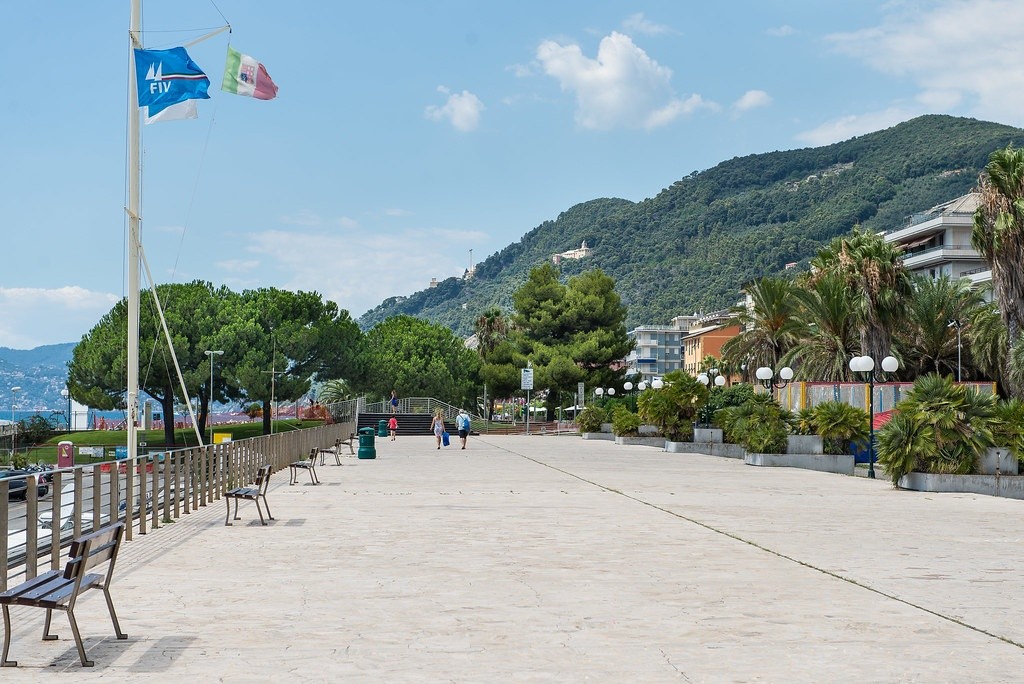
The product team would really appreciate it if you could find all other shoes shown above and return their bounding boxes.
[436,447,440,449]
[391,438,395,441]
[461,447,466,449]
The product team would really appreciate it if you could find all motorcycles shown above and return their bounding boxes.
[21,459,55,482]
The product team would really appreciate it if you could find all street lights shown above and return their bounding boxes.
[849,356,898,479]
[204,351,224,443]
[755,366,793,401]
[624,382,646,414]
[61,385,74,434]
[948,319,961,382]
[10,386,21,453]
[698,369,725,428]
[595,387,615,406]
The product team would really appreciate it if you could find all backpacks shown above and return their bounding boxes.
[459,414,470,431]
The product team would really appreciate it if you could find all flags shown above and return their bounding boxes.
[221,45,279,100]
[134,46,211,126]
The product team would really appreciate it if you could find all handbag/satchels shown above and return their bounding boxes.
[443,432,450,446]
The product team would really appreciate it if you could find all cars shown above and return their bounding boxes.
[0,470,49,501]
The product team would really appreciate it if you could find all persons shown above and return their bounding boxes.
[388,414,398,441]
[430,410,446,449]
[388,389,398,413]
[308,398,313,406]
[455,408,472,449]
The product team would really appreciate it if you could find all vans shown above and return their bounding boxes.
[38,512,108,529]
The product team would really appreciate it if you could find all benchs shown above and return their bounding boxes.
[224,464,270,526]
[0,520,125,667]
[289,447,319,485]
[339,432,355,455]
[320,439,341,466]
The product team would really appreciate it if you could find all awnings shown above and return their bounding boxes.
[907,231,945,249]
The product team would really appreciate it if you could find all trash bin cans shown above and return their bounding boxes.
[358,427,377,460]
[378,420,388,437]
